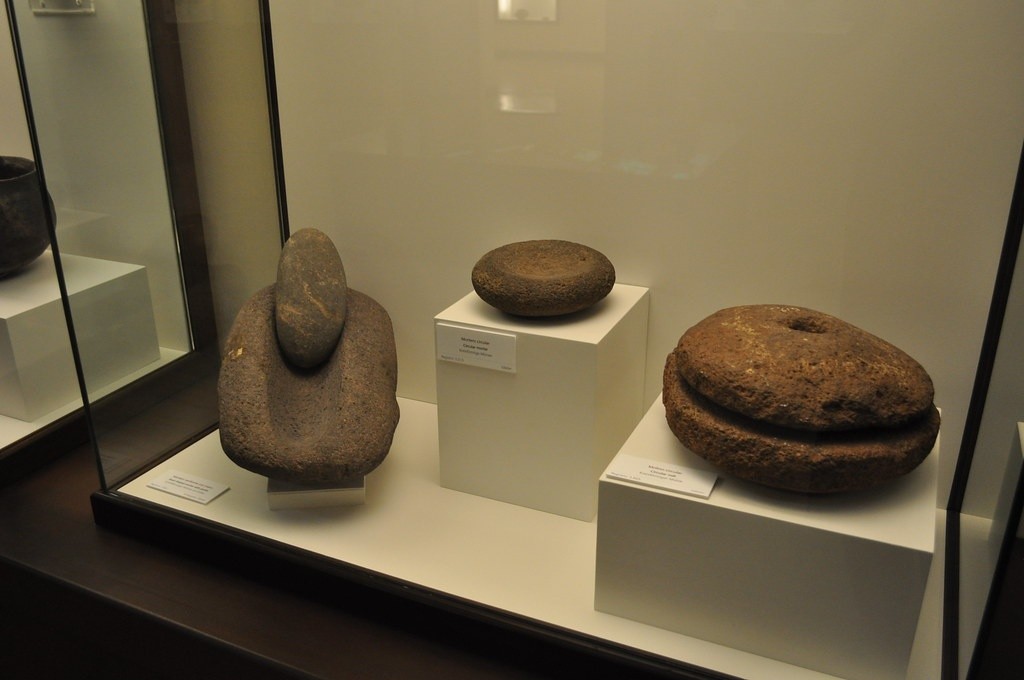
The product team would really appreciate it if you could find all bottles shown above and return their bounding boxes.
[0,156,56,281]
